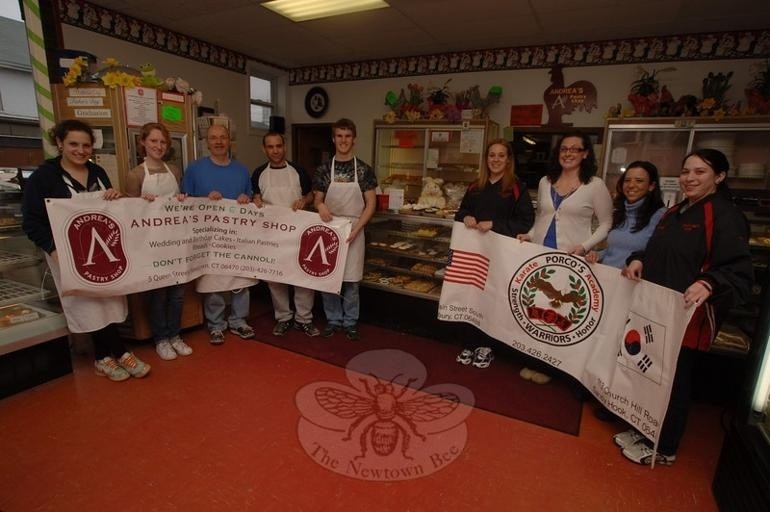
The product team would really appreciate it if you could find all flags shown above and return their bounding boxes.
[443,248,490,292]
[616,310,666,385]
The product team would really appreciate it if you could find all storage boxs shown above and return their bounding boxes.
[0,227,77,402]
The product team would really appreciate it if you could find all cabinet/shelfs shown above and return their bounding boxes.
[595,113,769,211]
[370,117,501,204]
[361,208,455,305]
[51,77,206,346]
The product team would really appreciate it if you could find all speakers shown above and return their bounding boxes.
[270,116,285,134]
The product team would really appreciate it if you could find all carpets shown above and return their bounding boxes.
[238,307,584,439]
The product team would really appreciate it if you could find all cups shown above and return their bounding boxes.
[377,194,389,211]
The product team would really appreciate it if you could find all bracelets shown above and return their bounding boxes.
[696,279,712,297]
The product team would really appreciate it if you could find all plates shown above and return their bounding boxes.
[304,86,331,119]
[696,132,770,176]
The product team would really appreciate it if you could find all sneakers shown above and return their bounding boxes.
[613,427,676,466]
[95,359,129,382]
[321,320,342,338]
[519,366,552,384]
[208,320,225,345]
[156,338,177,361]
[472,347,495,370]
[171,335,193,356]
[344,317,360,341]
[119,351,151,378]
[294,313,320,338]
[273,312,292,337]
[229,318,256,339]
[456,344,471,366]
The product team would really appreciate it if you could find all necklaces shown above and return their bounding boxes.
[553,178,579,211]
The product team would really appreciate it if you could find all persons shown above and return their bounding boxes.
[18,119,152,384]
[612,147,754,468]
[180,123,256,346]
[251,130,321,338]
[515,130,613,385]
[453,138,537,370]
[123,122,193,362]
[584,160,671,279]
[310,117,378,340]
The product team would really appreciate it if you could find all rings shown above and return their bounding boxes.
[586,255,589,257]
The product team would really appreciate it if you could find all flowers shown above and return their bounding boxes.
[61,55,143,91]
[378,77,483,127]
[613,61,770,123]
